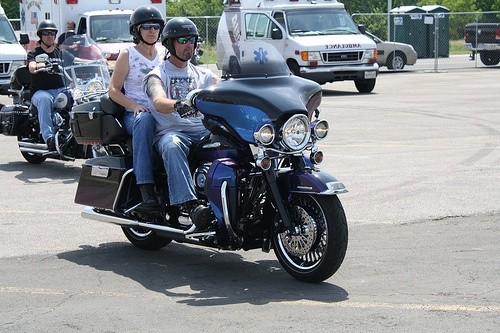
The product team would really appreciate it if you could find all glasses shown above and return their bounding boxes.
[172,36,195,44]
[41,31,56,36]
[140,23,161,30]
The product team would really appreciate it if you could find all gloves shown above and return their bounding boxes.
[174,100,195,119]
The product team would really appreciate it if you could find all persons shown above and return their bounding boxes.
[58,20,80,87]
[142,17,234,231]
[108,5,171,206]
[27,20,109,151]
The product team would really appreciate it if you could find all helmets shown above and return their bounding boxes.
[37,20,58,33]
[161,17,199,45]
[129,5,165,29]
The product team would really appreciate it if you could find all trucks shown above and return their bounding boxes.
[18,0,167,75]
[0,6,34,94]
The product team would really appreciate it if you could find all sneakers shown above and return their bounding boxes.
[189,204,210,231]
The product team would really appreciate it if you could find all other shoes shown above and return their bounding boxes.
[47,138,56,150]
[140,185,158,204]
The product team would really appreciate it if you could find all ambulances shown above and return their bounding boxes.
[215,0,377,93]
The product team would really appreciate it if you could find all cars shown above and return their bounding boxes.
[359,25,418,72]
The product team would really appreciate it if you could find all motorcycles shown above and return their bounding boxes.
[70,39,349,284]
[1,35,125,163]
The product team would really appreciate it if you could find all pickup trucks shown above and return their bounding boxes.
[464,12,500,67]
